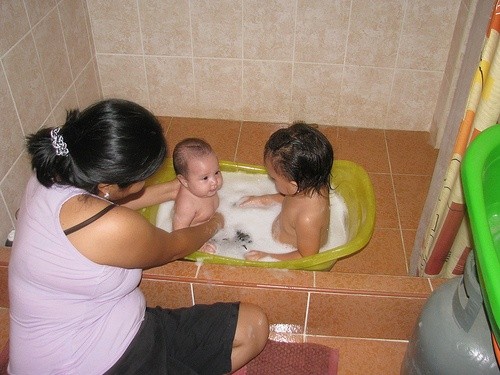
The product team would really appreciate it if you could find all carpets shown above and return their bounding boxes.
[230,339,339,375]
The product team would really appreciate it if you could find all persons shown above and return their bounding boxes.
[172,137,224,256]
[234,123,335,262]
[6,98,269,375]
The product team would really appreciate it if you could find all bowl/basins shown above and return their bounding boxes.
[460,124,500,346]
